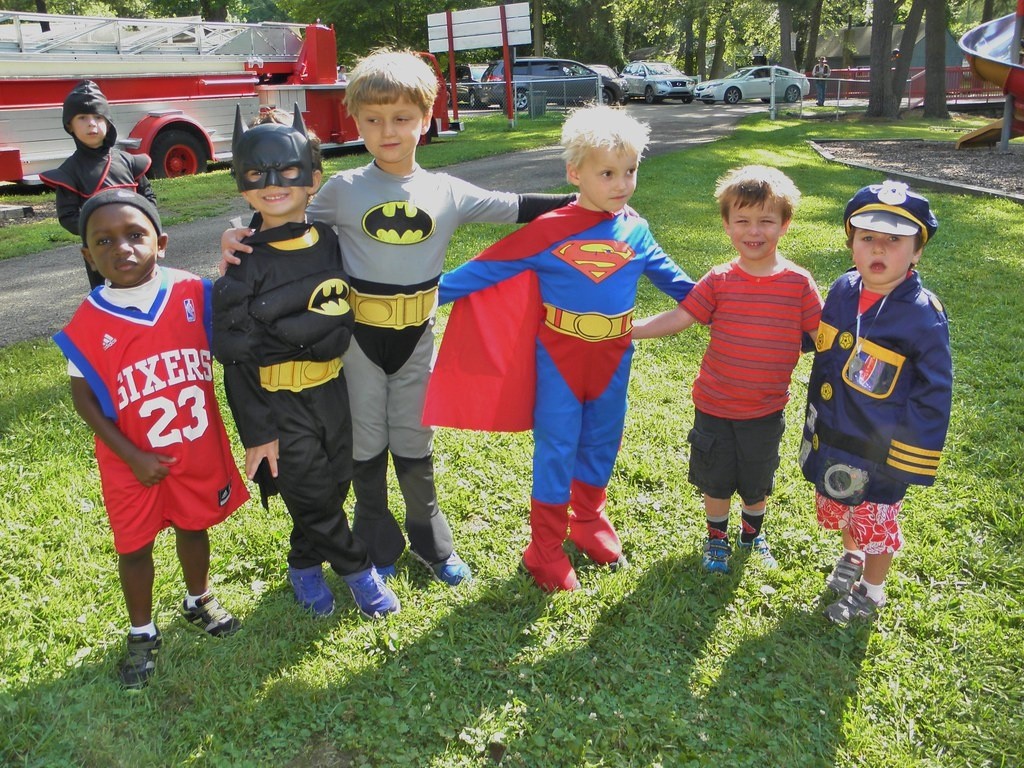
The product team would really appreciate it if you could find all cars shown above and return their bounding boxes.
[694,65,810,104]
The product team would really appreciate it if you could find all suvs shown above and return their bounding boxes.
[619,60,695,104]
[445,57,631,111]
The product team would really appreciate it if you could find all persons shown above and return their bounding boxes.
[800,180,953,628]
[440,106,695,593]
[220,47,580,591]
[210,102,399,622]
[632,164,823,577]
[39,80,157,291]
[51,189,251,689]
[812,56,831,106]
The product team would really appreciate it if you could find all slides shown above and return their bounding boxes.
[955,0,1024,151]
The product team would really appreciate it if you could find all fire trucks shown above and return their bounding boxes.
[0,10,458,186]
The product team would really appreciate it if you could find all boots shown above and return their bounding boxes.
[522,497,580,595]
[569,477,622,564]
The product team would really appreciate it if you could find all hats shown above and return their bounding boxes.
[843,181,938,246]
[79,188,161,248]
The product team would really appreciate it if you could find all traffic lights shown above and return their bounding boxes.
[893,49,899,59]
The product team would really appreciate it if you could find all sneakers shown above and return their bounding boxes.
[822,582,886,629]
[342,560,401,622]
[373,562,408,601]
[288,563,336,618]
[703,536,731,578]
[180,592,243,639]
[121,623,164,694]
[409,541,472,590]
[737,524,778,572]
[825,552,864,598]
